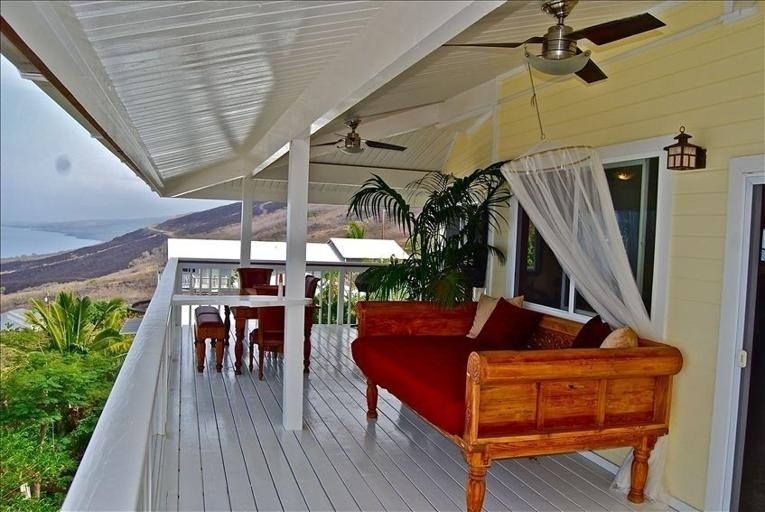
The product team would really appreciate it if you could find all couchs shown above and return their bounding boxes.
[350,301,682,512]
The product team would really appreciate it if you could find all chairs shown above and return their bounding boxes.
[237,268,274,340]
[304,275,320,373]
[249,307,283,380]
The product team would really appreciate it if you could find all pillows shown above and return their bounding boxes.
[466,293,638,349]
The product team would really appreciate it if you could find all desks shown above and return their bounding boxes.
[218,288,258,374]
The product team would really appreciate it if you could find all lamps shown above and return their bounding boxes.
[523,43,592,76]
[338,143,364,155]
[663,125,700,170]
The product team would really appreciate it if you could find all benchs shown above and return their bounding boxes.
[194,305,226,372]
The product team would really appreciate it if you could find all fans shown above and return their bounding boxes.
[310,117,407,151]
[441,0,667,83]
